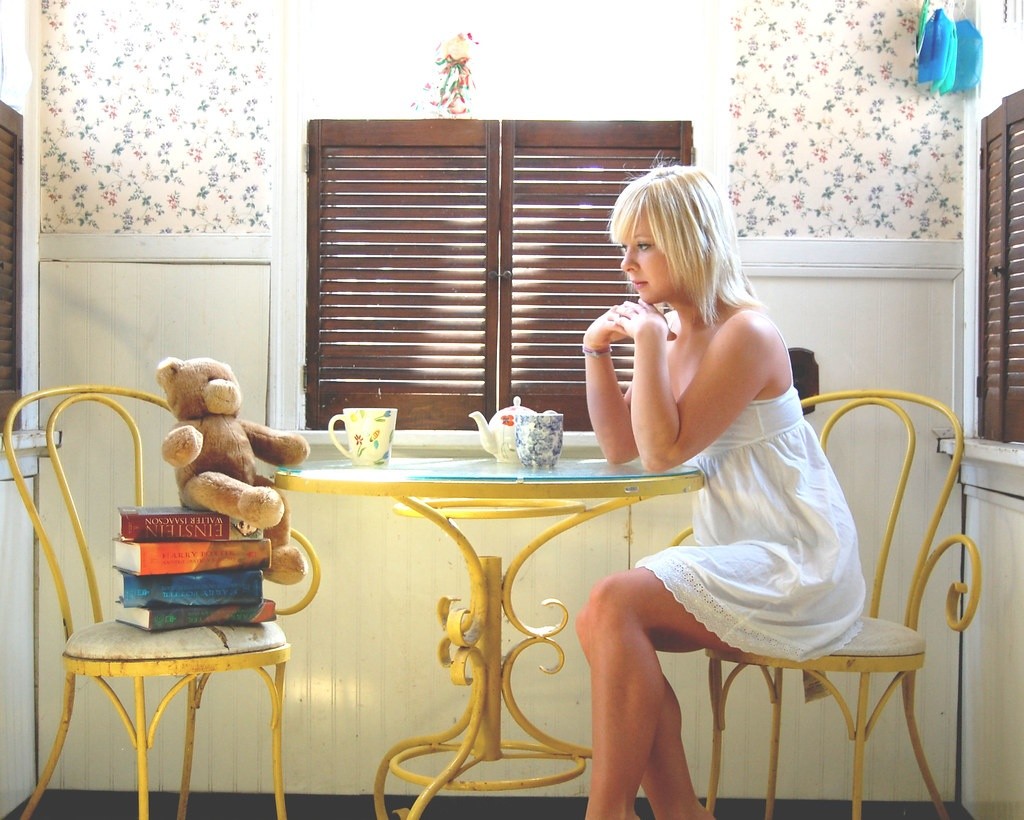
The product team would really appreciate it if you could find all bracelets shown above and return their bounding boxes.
[581,345,613,358]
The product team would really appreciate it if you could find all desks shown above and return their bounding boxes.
[275,459,705,820]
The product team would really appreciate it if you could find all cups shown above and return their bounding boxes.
[329,408,397,467]
[515,413,563,466]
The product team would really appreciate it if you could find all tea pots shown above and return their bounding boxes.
[468,396,538,462]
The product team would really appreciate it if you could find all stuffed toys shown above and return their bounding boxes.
[154,357,310,585]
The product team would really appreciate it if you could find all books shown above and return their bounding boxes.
[110,505,277,633]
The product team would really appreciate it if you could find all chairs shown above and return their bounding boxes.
[4,384,321,820]
[705,390,981,820]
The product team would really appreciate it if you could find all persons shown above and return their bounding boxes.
[574,163,866,818]
